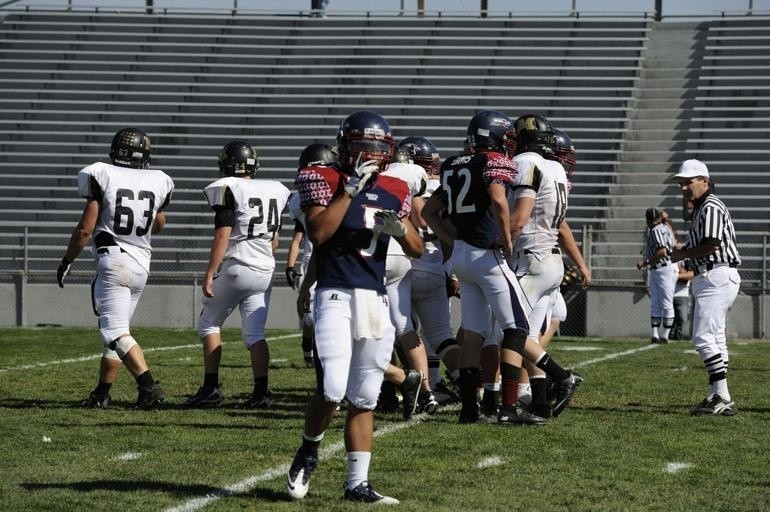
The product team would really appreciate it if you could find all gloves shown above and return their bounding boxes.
[287,268,302,290]
[344,152,379,198]
[374,210,407,237]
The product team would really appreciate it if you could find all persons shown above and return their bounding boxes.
[638,207,691,343]
[668,158,742,416]
[182,139,290,407]
[55,127,175,412]
[282,110,428,508]
[284,108,589,426]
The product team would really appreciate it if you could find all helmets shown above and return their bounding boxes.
[298,110,574,176]
[109,128,150,169]
[218,141,259,179]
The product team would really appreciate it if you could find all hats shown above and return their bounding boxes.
[646,208,660,217]
[669,158,709,181]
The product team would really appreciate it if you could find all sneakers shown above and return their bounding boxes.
[380,369,583,426]
[77,382,272,410]
[343,481,399,504]
[287,446,318,500]
[691,395,738,416]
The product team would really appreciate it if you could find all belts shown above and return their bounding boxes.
[693,263,736,275]
[97,248,125,254]
[523,248,560,254]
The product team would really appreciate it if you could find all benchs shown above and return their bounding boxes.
[2,6,770,289]
